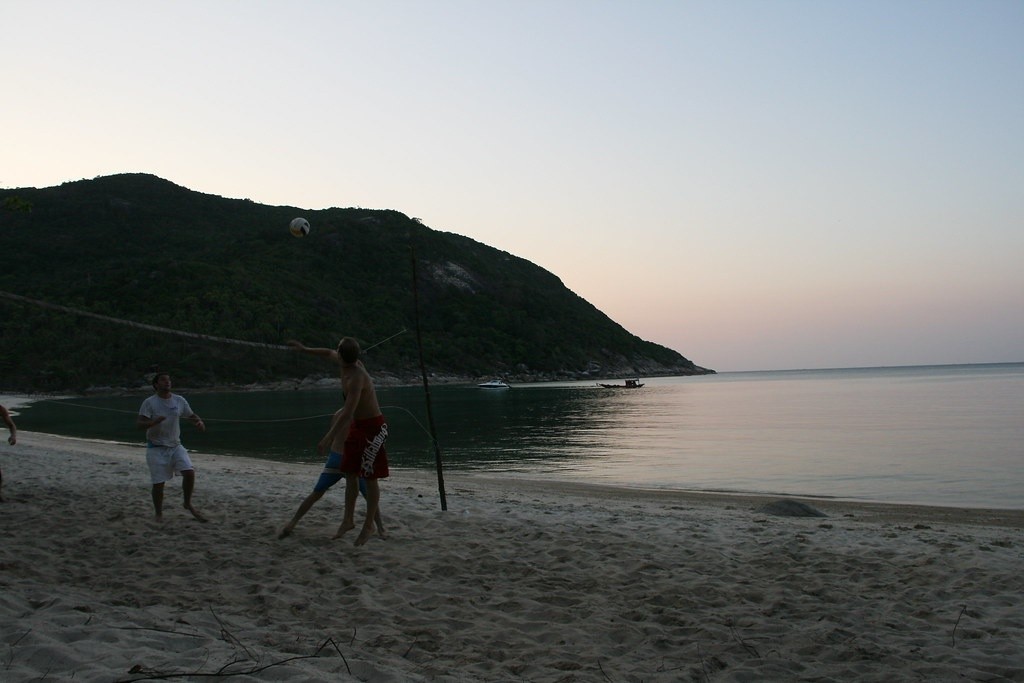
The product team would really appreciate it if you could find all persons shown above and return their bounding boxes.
[136,372,207,520]
[0,405,16,481]
[285,335,390,547]
[276,390,391,543]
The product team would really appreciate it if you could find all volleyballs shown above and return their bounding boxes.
[289,217,310,237]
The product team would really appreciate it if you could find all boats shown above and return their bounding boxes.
[477,379,513,389]
[595,378,646,389]
